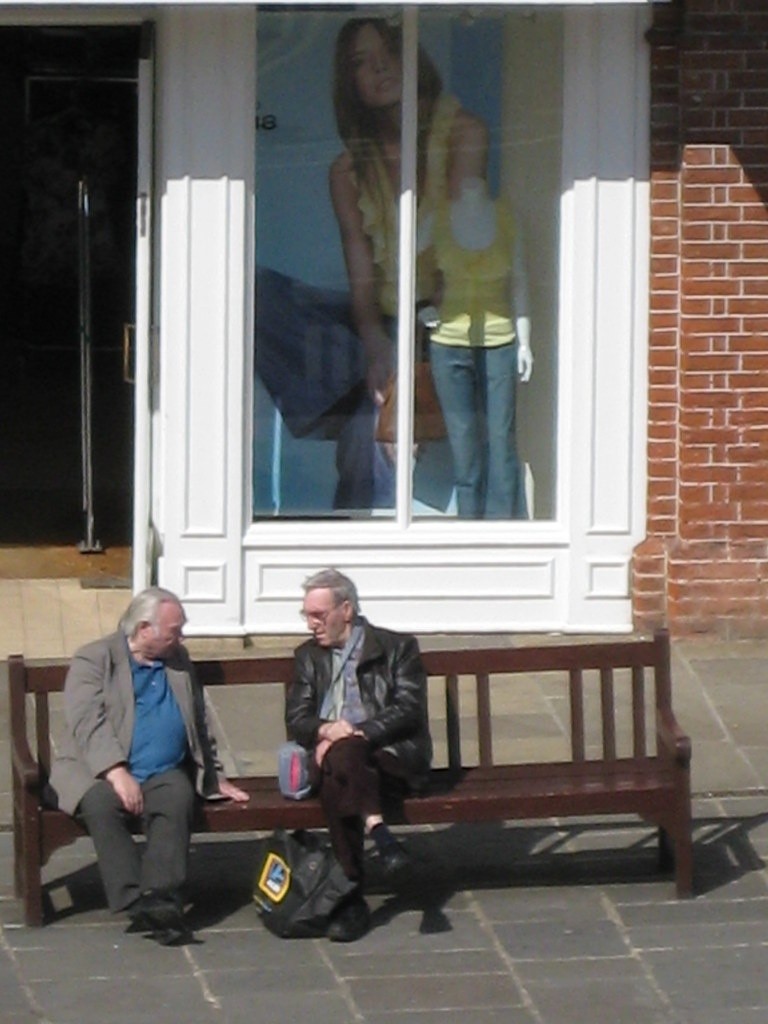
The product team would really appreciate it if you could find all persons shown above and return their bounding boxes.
[415,115,535,520]
[329,17,491,445]
[45,587,250,948]
[284,568,435,942]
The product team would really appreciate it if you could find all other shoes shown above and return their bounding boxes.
[375,833,407,872]
[328,894,368,941]
[128,892,189,944]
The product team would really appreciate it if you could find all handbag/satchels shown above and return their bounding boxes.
[252,825,358,937]
[278,737,325,802]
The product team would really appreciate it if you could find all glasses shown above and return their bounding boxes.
[300,597,347,626]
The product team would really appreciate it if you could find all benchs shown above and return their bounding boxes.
[7,626,693,928]
